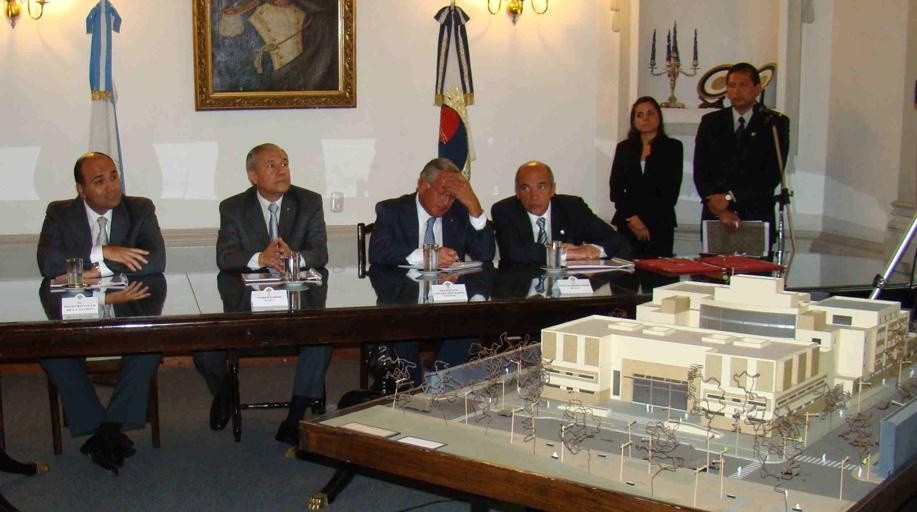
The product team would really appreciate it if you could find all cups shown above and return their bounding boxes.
[283,250,300,281]
[65,257,84,287]
[545,240,561,268]
[422,242,437,271]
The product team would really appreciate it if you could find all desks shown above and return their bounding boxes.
[1,249,915,364]
[295,343,916,511]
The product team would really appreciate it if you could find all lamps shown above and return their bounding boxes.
[486,0,551,26]
[0,0,49,28]
[650,21,700,107]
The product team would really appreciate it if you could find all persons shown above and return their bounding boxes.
[36,152,165,480]
[502,267,612,300]
[369,156,496,391]
[192,141,319,448]
[693,64,791,256]
[370,268,496,304]
[216,269,331,313]
[492,160,618,265]
[40,274,164,320]
[610,97,683,258]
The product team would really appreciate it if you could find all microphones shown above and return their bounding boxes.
[753,103,784,119]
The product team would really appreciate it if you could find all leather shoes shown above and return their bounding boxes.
[210,374,236,431]
[275,420,297,446]
[81,432,134,471]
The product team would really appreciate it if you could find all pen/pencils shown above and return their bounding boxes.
[434,244,461,262]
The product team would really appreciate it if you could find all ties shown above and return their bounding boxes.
[536,218,547,245]
[736,117,745,132]
[424,218,435,244]
[268,204,279,240]
[97,217,108,246]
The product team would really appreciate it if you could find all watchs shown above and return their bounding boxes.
[724,191,733,204]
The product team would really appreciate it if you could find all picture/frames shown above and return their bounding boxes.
[190,0,358,113]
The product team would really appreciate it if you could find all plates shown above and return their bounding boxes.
[417,269,441,277]
[62,288,89,292]
[539,266,566,273]
[279,278,307,286]
[279,285,309,292]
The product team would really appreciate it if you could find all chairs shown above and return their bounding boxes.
[40,353,162,457]
[357,221,387,387]
[227,346,328,445]
